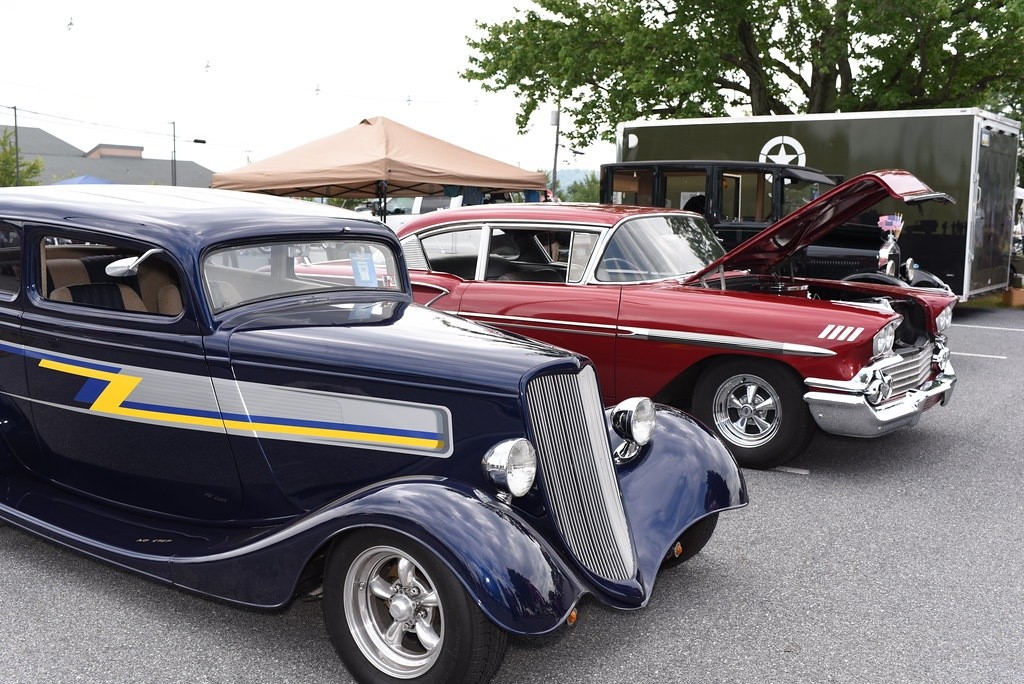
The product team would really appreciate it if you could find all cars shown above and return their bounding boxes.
[254,168,960,469]
[0,184,750,684]
[600,160,952,289]
[355,192,516,260]
[1010,251,1024,285]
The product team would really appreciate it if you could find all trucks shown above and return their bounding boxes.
[615,106,1022,302]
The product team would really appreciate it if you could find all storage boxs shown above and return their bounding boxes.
[1002,288,1024,307]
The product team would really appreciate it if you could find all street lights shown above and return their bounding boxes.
[551,144,584,197]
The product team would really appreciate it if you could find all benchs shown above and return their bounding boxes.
[44,253,142,300]
[426,254,523,280]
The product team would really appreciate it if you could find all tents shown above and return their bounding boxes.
[212,117,547,223]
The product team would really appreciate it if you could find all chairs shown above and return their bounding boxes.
[50,282,150,314]
[497,269,565,283]
[158,280,243,317]
